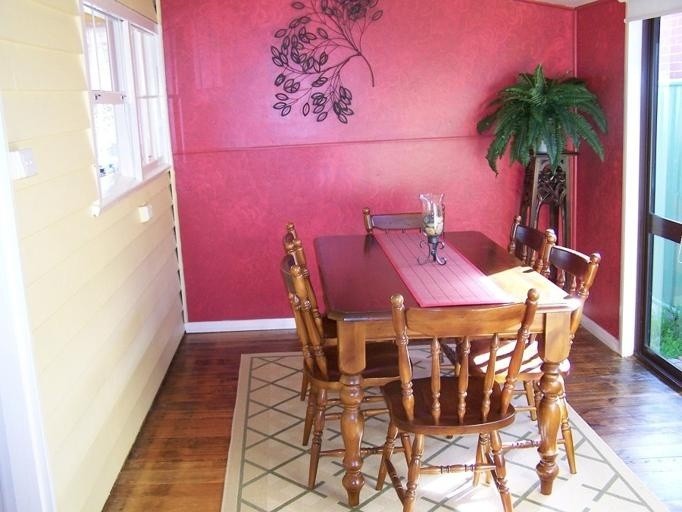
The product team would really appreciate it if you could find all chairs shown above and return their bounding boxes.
[376,288,540,512]
[504,216,555,274]
[284,242,414,489]
[362,204,445,234]
[473,234,601,486]
[282,223,338,400]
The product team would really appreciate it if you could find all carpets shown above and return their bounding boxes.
[219,343,667,512]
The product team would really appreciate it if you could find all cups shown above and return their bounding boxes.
[419,192,447,237]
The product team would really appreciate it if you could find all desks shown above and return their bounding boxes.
[312,231,582,506]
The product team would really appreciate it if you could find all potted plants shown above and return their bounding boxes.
[476,63,607,176]
